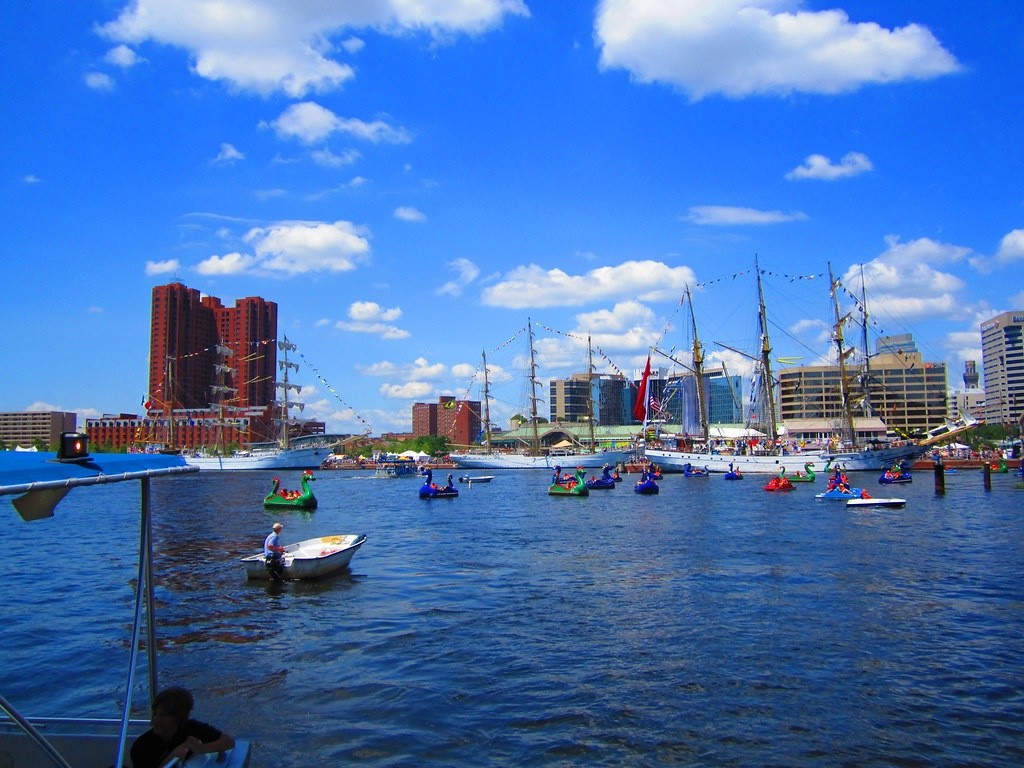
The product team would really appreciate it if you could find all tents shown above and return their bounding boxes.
[397,450,431,464]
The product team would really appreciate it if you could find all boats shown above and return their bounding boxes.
[240,534,368,580]
[878,468,912,485]
[724,461,744,480]
[126,333,373,472]
[764,477,796,492]
[457,474,496,484]
[823,460,847,473]
[638,250,985,474]
[780,461,816,483]
[814,463,862,500]
[376,452,418,478]
[547,461,711,497]
[443,316,639,470]
[263,469,318,510]
[845,498,907,507]
[418,467,459,501]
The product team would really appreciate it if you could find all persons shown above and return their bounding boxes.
[430,482,445,491]
[279,488,301,500]
[691,465,701,475]
[556,462,661,490]
[926,447,1003,459]
[263,523,285,588]
[729,458,741,476]
[129,688,234,768]
[884,458,910,479]
[826,472,852,494]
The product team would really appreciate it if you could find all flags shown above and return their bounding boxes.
[443,400,455,409]
[649,391,661,412]
[633,355,650,420]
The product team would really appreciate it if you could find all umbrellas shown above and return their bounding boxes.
[553,439,573,456]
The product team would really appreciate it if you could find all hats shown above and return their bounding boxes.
[273,523,284,530]
